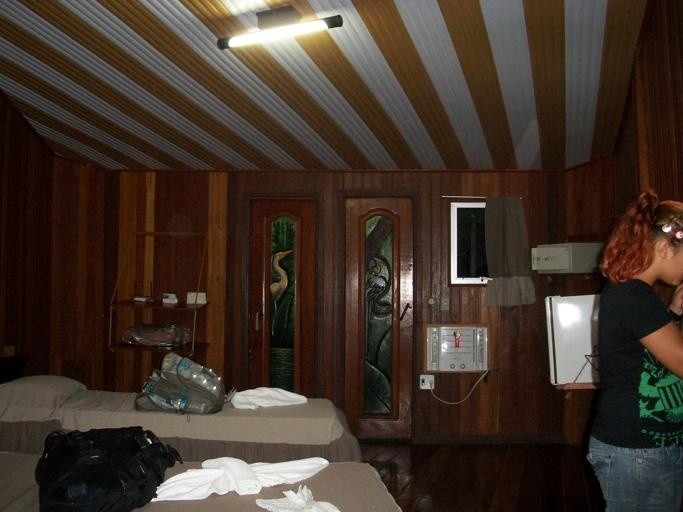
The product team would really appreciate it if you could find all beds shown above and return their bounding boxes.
[1,372,361,463]
[1,448,405,512]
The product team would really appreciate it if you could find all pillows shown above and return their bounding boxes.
[2,374,85,409]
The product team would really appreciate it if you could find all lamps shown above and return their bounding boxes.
[217,14,342,49]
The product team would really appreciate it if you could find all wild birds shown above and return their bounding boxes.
[269,247,293,336]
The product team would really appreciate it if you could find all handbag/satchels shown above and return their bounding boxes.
[35,426,183,512]
[135,351,225,414]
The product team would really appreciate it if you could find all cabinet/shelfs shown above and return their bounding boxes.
[106,232,211,355]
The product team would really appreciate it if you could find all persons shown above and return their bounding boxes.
[584,188,683,510]
